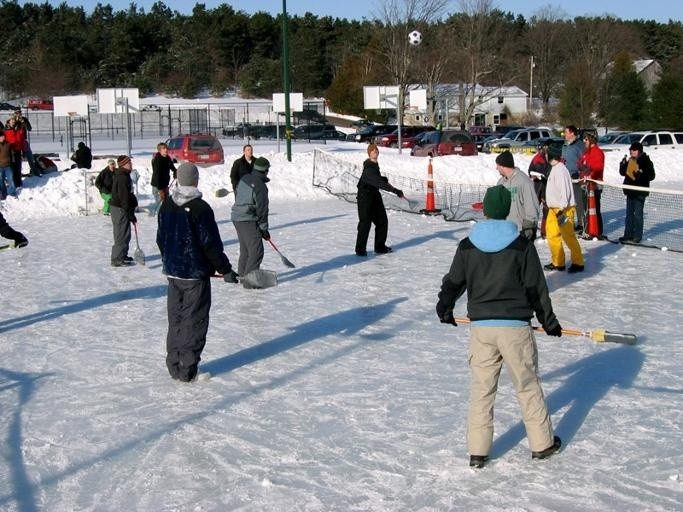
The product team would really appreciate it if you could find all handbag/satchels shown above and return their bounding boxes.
[29,154,56,174]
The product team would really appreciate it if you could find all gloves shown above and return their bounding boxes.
[546,323,562,337]
[223,270,239,284]
[261,230,270,241]
[439,309,458,327]
[524,229,533,240]
[158,190,165,201]
[126,211,136,225]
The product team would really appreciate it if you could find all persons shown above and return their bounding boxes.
[1,210,28,247]
[93,159,116,213]
[494,149,541,244]
[579,130,604,241]
[107,156,138,269]
[614,143,655,242]
[229,145,257,196]
[230,158,270,277]
[150,144,177,210]
[152,162,236,383]
[528,140,550,238]
[437,184,564,469]
[543,143,587,273]
[0,105,58,198]
[354,141,405,255]
[69,141,91,169]
[561,127,585,236]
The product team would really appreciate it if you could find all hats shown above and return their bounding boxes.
[367,144,378,156]
[628,142,643,153]
[116,155,130,167]
[107,160,115,165]
[13,107,21,114]
[495,150,514,168]
[483,185,511,219]
[534,138,562,157]
[176,163,198,186]
[583,129,598,142]
[254,157,269,171]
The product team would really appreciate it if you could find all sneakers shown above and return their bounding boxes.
[170,371,210,386]
[544,262,566,272]
[355,246,367,256]
[110,255,132,267]
[567,263,584,272]
[573,225,642,243]
[469,454,491,468]
[374,246,391,253]
[14,235,27,248]
[531,436,561,459]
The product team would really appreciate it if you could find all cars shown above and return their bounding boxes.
[142,104,161,111]
[346,120,477,156]
[221,111,346,142]
[0,103,19,111]
[469,125,683,153]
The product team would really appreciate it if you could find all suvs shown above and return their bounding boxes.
[29,100,53,110]
[152,131,224,168]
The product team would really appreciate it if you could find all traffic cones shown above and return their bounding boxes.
[587,179,603,240]
[419,159,441,213]
